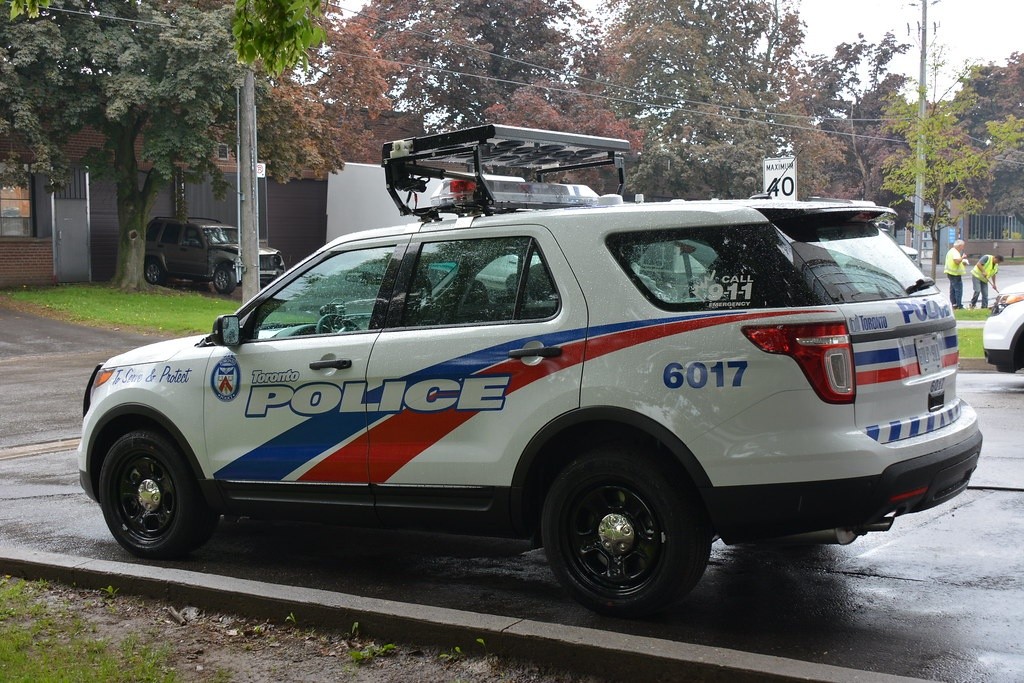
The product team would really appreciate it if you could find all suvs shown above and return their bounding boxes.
[75,122,988,623]
[143,215,285,295]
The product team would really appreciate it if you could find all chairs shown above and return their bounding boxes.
[463,281,490,305]
[406,274,442,324]
[187,229,197,240]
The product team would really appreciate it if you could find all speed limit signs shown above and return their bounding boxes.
[763,156,798,201]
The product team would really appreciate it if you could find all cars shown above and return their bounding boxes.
[423,240,720,303]
[981,281,1024,374]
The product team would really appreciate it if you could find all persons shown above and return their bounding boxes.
[943,239,967,309]
[969,254,1004,308]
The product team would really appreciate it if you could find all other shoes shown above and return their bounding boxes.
[953,305,963,309]
